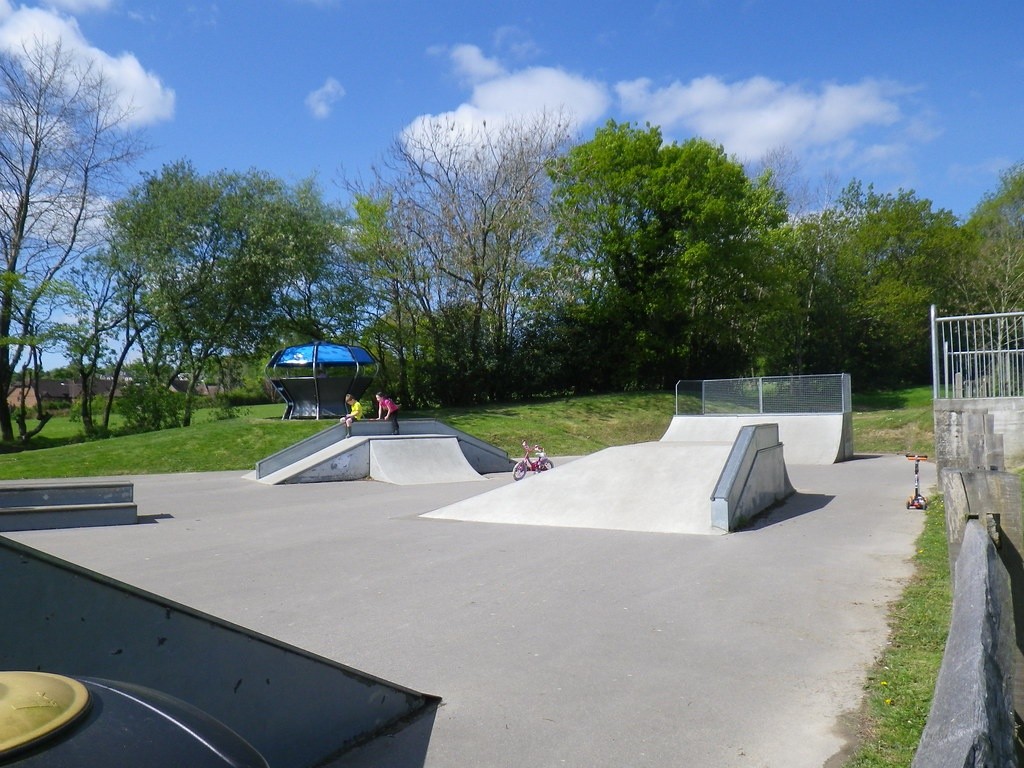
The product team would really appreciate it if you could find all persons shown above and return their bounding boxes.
[340,394,362,438]
[375,392,400,435]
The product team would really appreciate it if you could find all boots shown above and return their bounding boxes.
[346,426,352,438]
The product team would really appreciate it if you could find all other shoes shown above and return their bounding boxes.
[392,430,400,434]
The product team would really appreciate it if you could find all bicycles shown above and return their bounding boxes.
[512,439,555,482]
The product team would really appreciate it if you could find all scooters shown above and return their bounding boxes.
[905,452,928,510]
